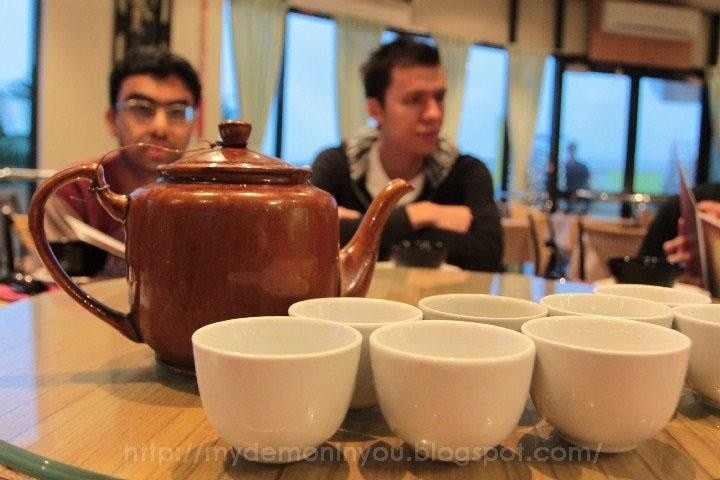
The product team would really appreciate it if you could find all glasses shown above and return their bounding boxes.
[115,101,197,123]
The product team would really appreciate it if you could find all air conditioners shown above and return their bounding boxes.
[601,1,702,40]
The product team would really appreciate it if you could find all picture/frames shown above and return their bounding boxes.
[113,1,175,68]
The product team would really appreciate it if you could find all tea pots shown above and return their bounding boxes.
[25,118,419,377]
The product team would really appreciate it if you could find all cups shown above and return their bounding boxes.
[673,302,719,405]
[520,314,695,456]
[287,295,424,413]
[538,292,676,330]
[417,292,550,333]
[191,315,365,466]
[593,283,712,309]
[366,319,535,465]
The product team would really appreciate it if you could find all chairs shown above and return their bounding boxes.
[574,188,676,284]
[498,191,557,278]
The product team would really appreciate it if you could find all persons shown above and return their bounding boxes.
[308,38,507,274]
[636,183,720,301]
[47,48,204,280]
[564,144,591,210]
